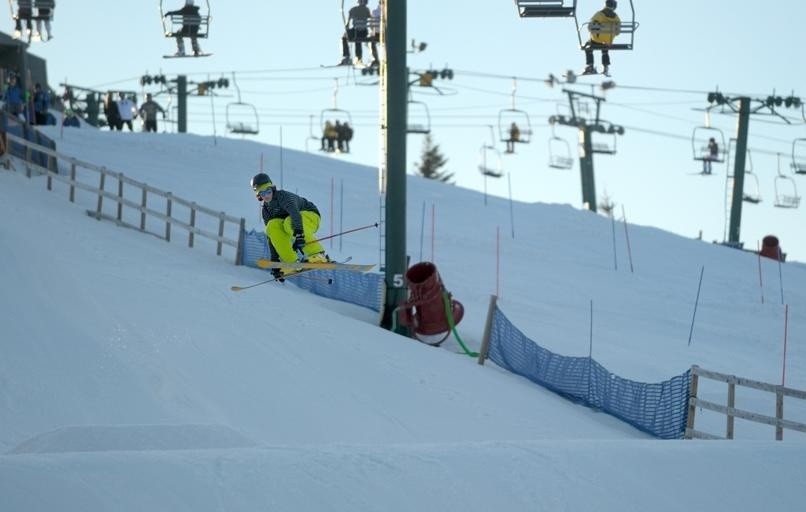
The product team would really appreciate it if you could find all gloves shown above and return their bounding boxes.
[270,267,286,283]
[291,233,305,256]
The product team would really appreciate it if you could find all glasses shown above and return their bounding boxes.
[259,188,273,198]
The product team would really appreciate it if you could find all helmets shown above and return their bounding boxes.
[251,172,276,201]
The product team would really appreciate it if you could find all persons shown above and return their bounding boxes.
[698,138,719,174]
[250,172,330,284]
[582,0,622,76]
[340,0,372,65]
[15,0,33,42]
[33,0,55,42]
[164,0,202,56]
[503,122,521,154]
[369,0,384,67]
[318,120,353,152]
[0,67,167,134]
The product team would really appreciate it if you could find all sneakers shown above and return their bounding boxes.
[279,268,302,276]
[584,65,609,73]
[304,254,328,263]
[340,58,379,65]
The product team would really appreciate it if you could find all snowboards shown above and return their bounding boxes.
[164,54,212,59]
